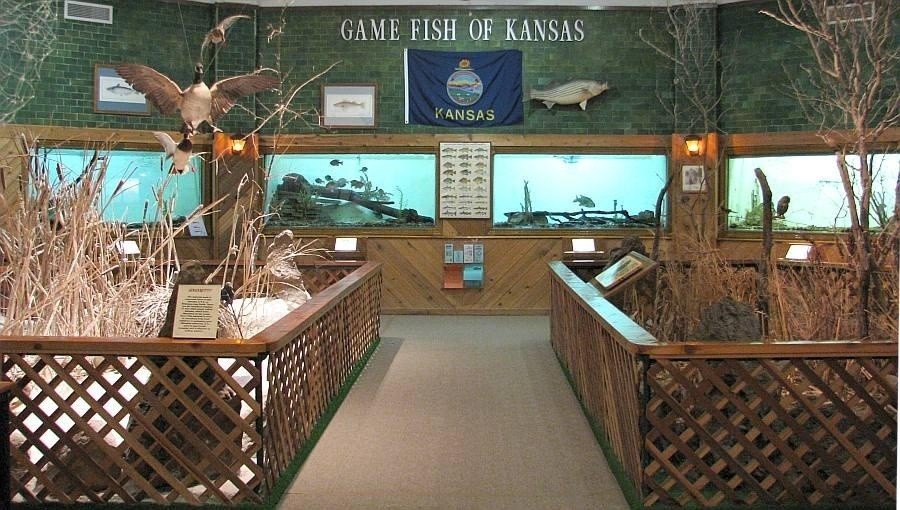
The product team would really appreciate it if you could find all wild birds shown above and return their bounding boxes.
[777,196,791,217]
[200,14,251,54]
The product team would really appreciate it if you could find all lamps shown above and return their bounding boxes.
[229,130,247,155]
[685,132,704,156]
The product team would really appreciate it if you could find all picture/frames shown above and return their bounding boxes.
[439,142,491,220]
[320,82,378,129]
[95,64,152,115]
[682,164,708,193]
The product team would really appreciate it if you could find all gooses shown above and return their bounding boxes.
[150,126,213,176]
[110,63,282,137]
